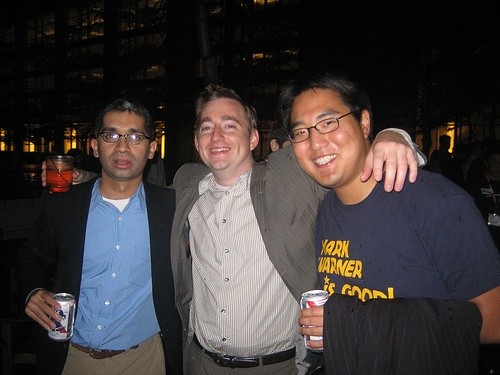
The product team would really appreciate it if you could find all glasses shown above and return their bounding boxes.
[286,110,357,143]
[98,132,150,145]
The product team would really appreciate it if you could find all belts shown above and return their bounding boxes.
[70,341,139,359]
[193,332,296,369]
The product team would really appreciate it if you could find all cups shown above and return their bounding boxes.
[46,155,74,193]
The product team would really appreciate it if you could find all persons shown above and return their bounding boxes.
[65,149,83,170]
[278,69,500,375]
[430,134,500,253]
[22,100,183,375]
[41,83,427,375]
[269,120,291,154]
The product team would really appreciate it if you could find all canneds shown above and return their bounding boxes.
[47,292,76,341]
[300,290,329,353]
